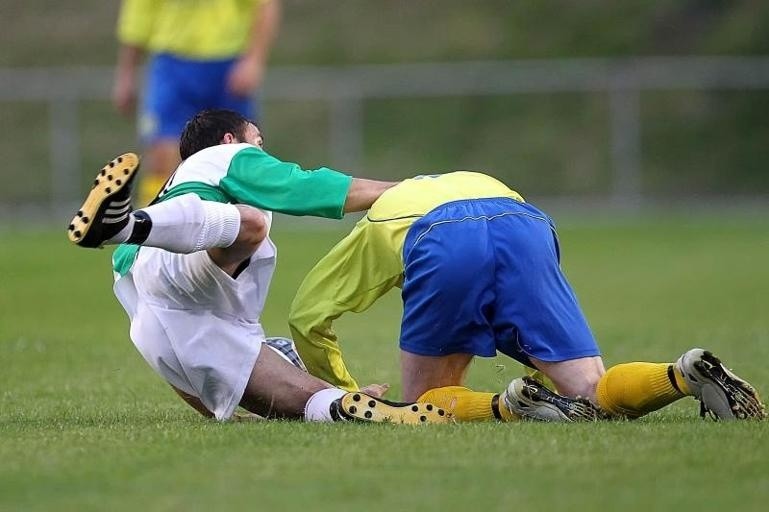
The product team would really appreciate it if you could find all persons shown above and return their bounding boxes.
[286,169,765,423]
[65,107,458,426]
[109,0,283,208]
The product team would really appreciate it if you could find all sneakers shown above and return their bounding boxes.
[675,348,769,422]
[330,392,453,424]
[504,376,611,421]
[67,151,140,250]
